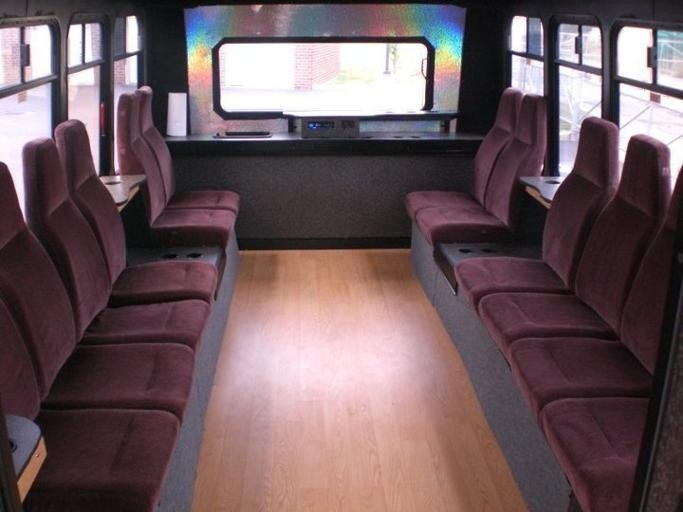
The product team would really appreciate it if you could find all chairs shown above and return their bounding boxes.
[137,86,240,214]
[117,94,237,248]
[403,86,523,224]
[417,93,548,246]
